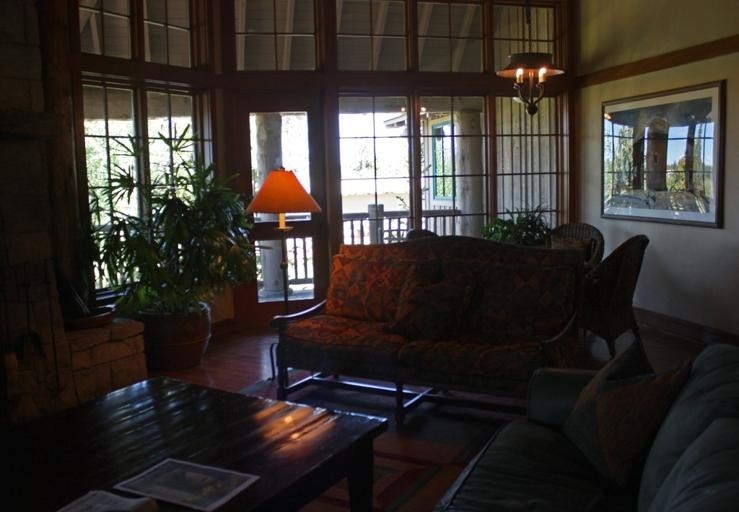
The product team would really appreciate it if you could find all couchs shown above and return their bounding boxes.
[269,235,581,430]
[433,339,738,512]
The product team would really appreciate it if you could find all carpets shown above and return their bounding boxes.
[237,365,478,512]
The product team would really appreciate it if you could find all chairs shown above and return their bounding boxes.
[547,221,650,358]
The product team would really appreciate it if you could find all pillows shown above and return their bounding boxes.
[555,337,693,484]
[386,265,474,341]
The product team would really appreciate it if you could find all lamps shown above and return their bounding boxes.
[247,171,323,318]
[495,0,564,115]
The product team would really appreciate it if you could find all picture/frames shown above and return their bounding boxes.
[600,79,728,230]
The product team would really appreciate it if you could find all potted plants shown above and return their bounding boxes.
[85,125,258,371]
